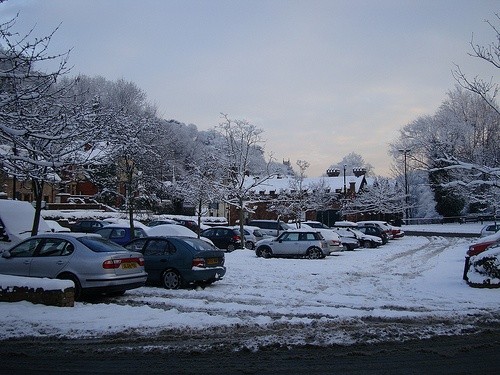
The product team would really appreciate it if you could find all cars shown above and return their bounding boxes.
[389,221,405,238]
[481,224,500,238]
[356,226,389,245]
[199,227,245,253]
[467,246,500,288]
[228,225,269,250]
[122,235,227,290]
[0,233,148,301]
[147,219,181,228]
[255,230,329,259]
[466,229,500,258]
[69,219,107,233]
[330,231,359,251]
[301,221,330,229]
[316,230,344,256]
[172,219,200,234]
[331,228,383,248]
[92,224,149,246]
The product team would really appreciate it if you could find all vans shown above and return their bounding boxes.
[249,220,297,238]
[357,221,394,239]
[334,221,357,229]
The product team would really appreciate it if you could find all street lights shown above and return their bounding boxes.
[398,148,410,225]
[341,164,348,221]
